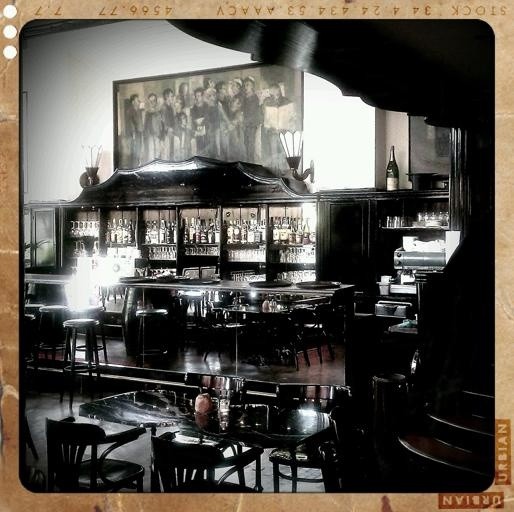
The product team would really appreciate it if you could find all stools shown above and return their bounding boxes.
[133,307,172,373]
[23,300,44,373]
[60,319,98,407]
[35,303,74,369]
[83,306,108,365]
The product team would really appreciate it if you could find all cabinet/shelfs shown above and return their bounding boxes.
[409,195,455,261]
[56,200,321,282]
[319,195,407,291]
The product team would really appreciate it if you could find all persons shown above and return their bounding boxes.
[226,77,243,162]
[125,92,143,171]
[263,84,295,164]
[202,88,221,160]
[239,76,261,160]
[169,97,188,157]
[158,88,176,161]
[178,83,194,149]
[215,82,231,157]
[142,93,164,164]
[189,88,208,155]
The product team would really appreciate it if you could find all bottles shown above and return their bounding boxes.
[180,218,185,244]
[105,220,111,243]
[277,216,282,229]
[111,218,117,242]
[287,216,296,245]
[207,218,215,244]
[123,217,136,244]
[385,145,399,192]
[140,218,177,244]
[200,218,208,244]
[184,216,189,244]
[295,217,304,244]
[117,218,123,243]
[280,217,288,240]
[189,217,196,244]
[195,216,201,244]
[269,216,274,240]
[272,216,280,241]
[302,218,310,244]
[221,218,267,244]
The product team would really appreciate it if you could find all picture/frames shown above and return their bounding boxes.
[112,62,305,179]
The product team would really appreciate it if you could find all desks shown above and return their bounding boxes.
[213,305,262,360]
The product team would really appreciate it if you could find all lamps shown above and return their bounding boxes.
[278,129,315,185]
[77,145,105,191]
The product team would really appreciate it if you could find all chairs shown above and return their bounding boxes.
[271,383,349,494]
[149,432,264,494]
[45,417,147,492]
[285,304,326,369]
[173,371,246,494]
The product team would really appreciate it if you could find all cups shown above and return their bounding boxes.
[393,217,401,228]
[386,216,394,227]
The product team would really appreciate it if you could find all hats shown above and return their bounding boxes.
[232,79,242,89]
[247,75,255,83]
